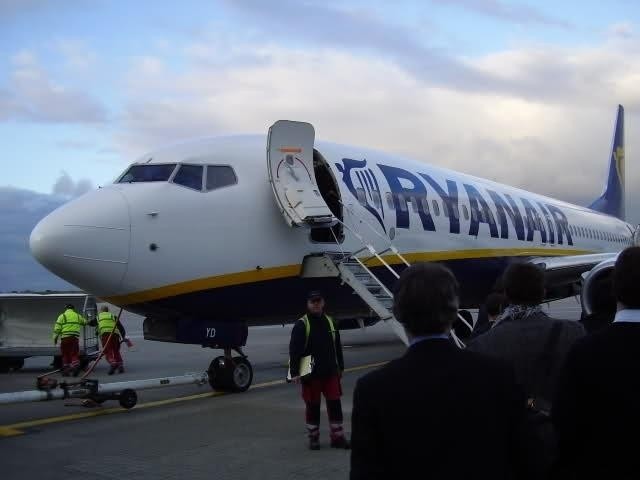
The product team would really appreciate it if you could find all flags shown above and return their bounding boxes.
[589,105,625,221]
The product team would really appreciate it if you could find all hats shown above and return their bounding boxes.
[304,291,325,300]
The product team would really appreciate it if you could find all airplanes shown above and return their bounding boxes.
[28,102,639,394]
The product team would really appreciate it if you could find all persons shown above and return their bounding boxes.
[460,259,587,480]
[552,245,640,479]
[86,304,127,374]
[52,302,88,377]
[471,293,509,339]
[347,259,539,479]
[289,285,355,451]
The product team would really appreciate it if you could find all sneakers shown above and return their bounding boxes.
[107,363,126,375]
[61,365,81,377]
[308,438,354,452]
